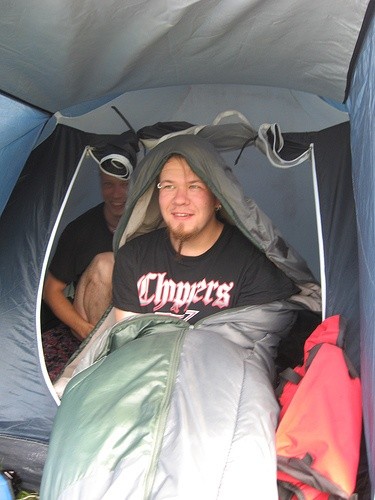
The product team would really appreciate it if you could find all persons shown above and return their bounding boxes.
[44,171,130,346]
[112,151,300,332]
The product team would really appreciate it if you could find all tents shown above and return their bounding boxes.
[0,0,373,500]
